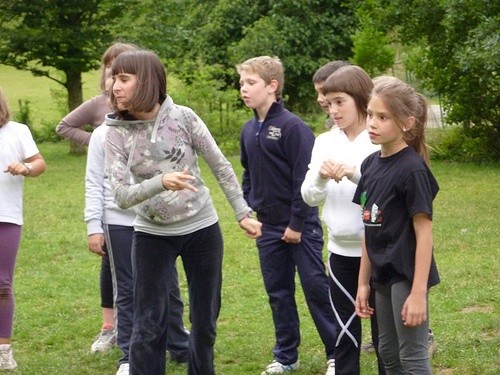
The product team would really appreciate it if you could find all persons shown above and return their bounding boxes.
[103,50,262,375]
[235,54,339,375]
[84,76,191,375]
[312,58,348,112]
[54,41,142,355]
[0,89,50,370]
[299,64,387,375]
[351,77,441,374]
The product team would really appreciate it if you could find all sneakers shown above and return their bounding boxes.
[91,325,116,352]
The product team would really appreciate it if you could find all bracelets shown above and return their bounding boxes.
[22,162,32,177]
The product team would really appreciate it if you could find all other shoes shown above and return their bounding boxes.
[116,363,130,375]
[324,359,336,375]
[261,358,299,375]
[0,345,18,371]
